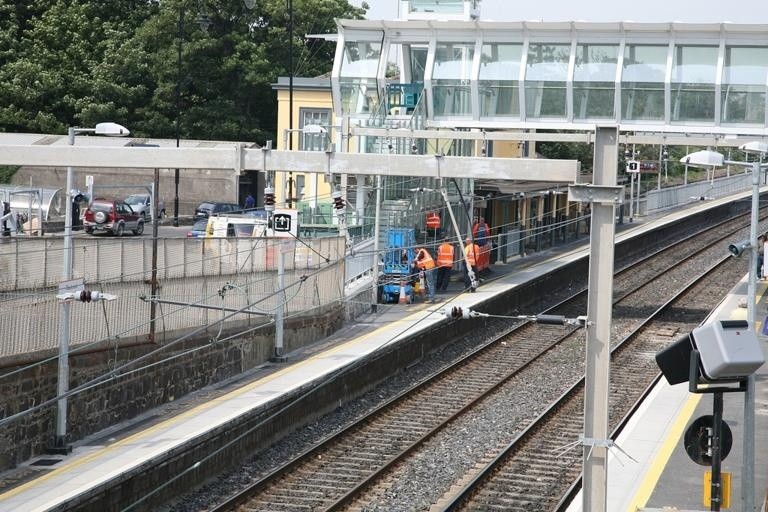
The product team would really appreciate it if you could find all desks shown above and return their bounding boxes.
[51,122,130,456]
[679,149,761,512]
[270,124,328,363]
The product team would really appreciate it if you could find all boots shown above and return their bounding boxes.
[81,198,144,236]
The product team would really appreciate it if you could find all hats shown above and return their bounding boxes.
[186,201,274,247]
[122,193,167,236]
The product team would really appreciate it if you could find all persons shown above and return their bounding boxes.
[756,232,766,282]
[436,235,455,292]
[473,217,492,272]
[460,236,481,294]
[243,191,255,212]
[412,244,437,304]
